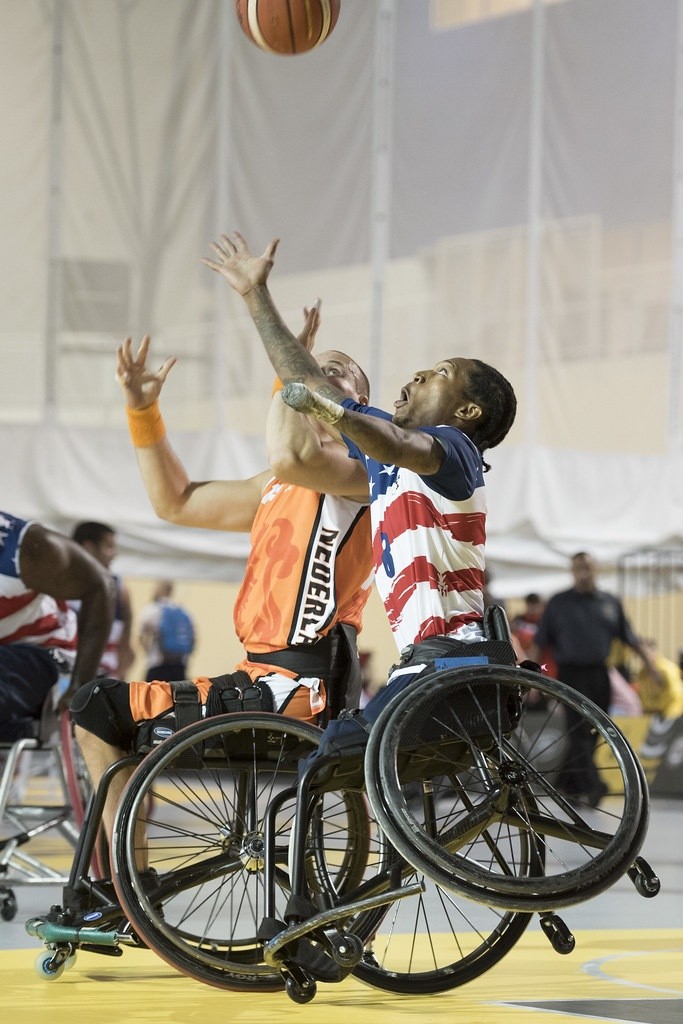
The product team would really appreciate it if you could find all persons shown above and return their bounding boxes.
[0,227,682,948]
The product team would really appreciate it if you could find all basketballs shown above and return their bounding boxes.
[234,0,342,56]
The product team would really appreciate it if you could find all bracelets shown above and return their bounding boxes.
[126,399,167,447]
[271,376,283,398]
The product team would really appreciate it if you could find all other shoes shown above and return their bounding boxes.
[588,783,607,807]
[141,867,164,919]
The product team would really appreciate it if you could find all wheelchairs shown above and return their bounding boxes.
[0,688,111,924]
[24,619,400,995]
[255,604,664,1007]
